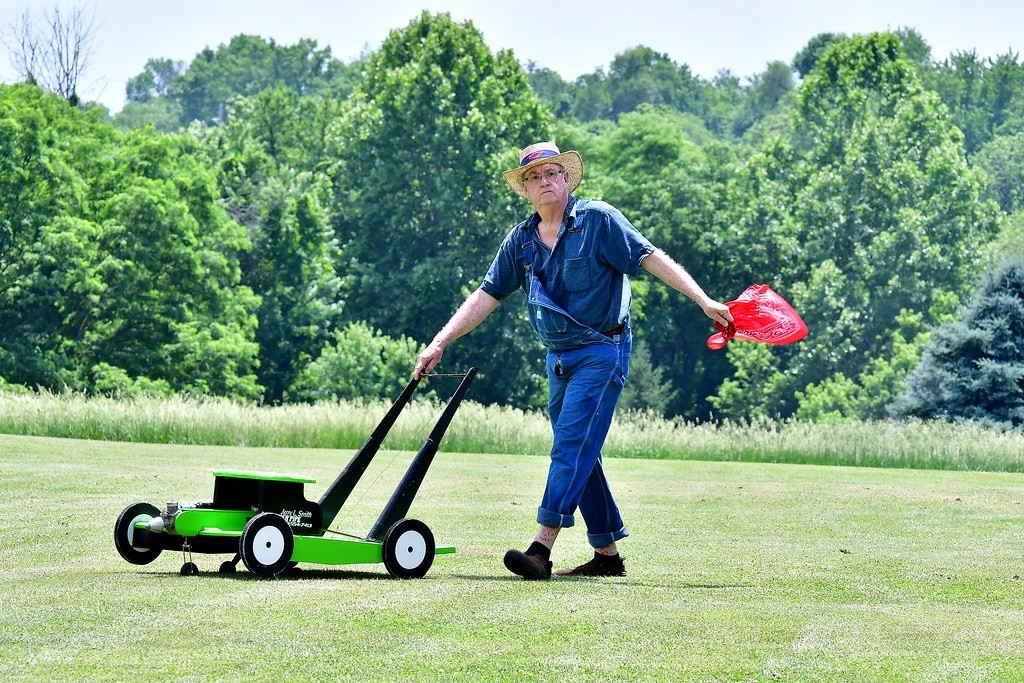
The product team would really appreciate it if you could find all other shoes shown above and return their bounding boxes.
[504,549,553,580]
[556,553,627,577]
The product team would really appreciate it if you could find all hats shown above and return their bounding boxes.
[502,142,583,200]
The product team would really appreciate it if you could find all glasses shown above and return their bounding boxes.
[523,170,566,183]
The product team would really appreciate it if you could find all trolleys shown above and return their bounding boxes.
[114,363,479,580]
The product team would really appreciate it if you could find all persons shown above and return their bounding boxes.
[414,143,734,580]
